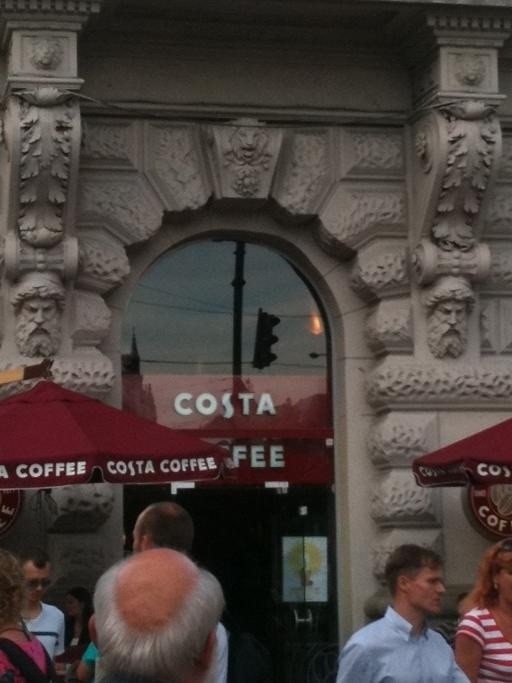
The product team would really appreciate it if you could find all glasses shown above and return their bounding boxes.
[27,578,53,589]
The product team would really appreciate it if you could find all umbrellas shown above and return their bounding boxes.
[1,379,234,490]
[412,418,512,487]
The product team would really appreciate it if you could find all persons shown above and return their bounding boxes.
[18,554,65,663]
[55,585,93,683]
[421,275,478,358]
[86,546,227,682]
[132,500,231,682]
[73,640,100,682]
[451,535,512,682]
[12,273,69,357]
[333,543,471,683]
[0,548,59,681]
[434,590,470,646]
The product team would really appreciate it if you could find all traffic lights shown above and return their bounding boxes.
[254,313,280,369]
